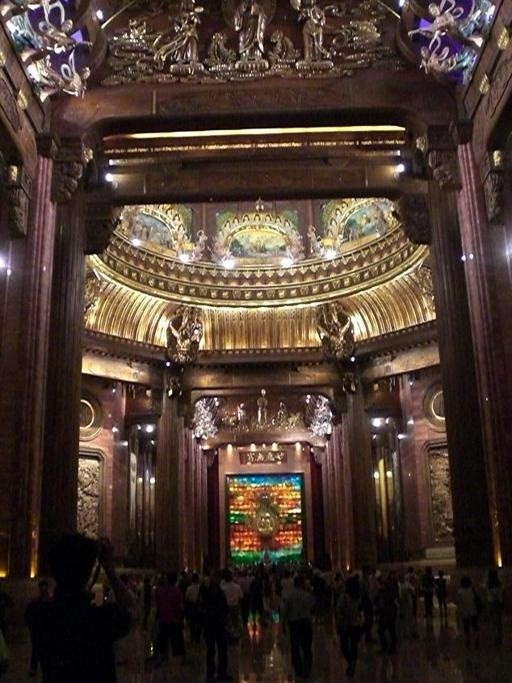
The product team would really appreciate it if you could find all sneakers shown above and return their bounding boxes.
[403,622,448,639]
[144,656,193,667]
[378,647,397,654]
[207,673,233,683]
[464,638,503,649]
[26,671,38,678]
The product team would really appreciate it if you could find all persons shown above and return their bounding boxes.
[228,0,269,61]
[25,57,91,102]
[2,533,135,681]
[254,388,271,426]
[457,569,504,650]
[12,17,95,68]
[119,559,450,681]
[296,0,325,65]
[317,317,358,393]
[166,319,202,397]
[405,2,481,57]
[151,0,205,75]
[413,40,475,79]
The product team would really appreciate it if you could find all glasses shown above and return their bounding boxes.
[40,588,47,591]
